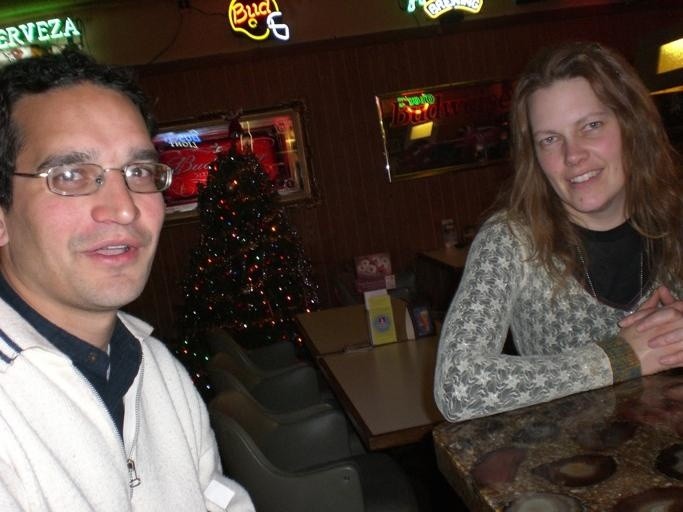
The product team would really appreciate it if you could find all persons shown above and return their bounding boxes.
[0,39,263,512]
[433,34,680,425]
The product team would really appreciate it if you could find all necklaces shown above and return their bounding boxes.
[571,228,644,305]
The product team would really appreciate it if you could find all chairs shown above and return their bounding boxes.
[197,325,370,512]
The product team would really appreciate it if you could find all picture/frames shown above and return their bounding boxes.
[147,104,328,230]
[372,78,521,184]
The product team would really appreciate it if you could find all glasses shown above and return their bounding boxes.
[12,161,174,196]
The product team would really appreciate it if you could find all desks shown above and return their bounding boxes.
[296,298,449,357]
[431,367,683,511]
[317,331,443,450]
